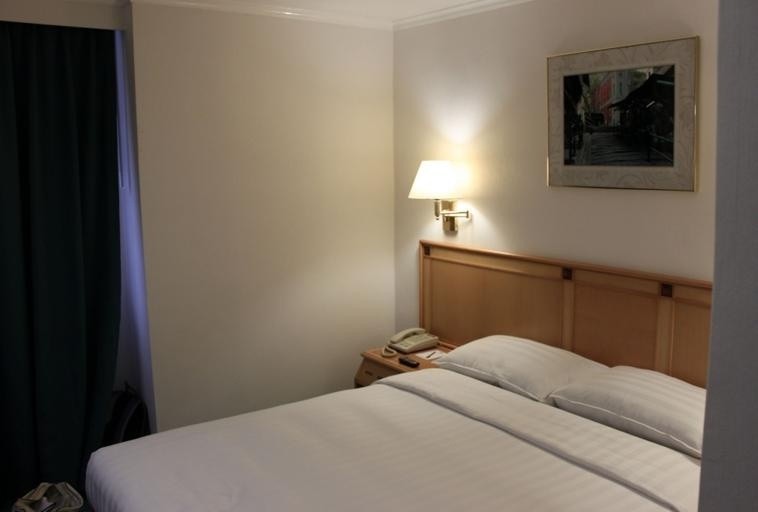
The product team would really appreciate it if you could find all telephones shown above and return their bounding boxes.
[387,327,439,353]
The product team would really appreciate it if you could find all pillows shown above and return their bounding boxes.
[548,365,707,459]
[429,334,610,407]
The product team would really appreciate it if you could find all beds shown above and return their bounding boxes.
[83,239,715,512]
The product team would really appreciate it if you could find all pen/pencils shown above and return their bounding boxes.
[427,352,435,358]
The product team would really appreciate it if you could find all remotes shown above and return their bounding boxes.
[399,356,419,367]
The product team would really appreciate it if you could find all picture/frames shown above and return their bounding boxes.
[546,36,701,192]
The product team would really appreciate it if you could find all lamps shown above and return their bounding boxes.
[407,160,469,233]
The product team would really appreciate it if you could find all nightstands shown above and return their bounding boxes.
[354,345,452,388]
